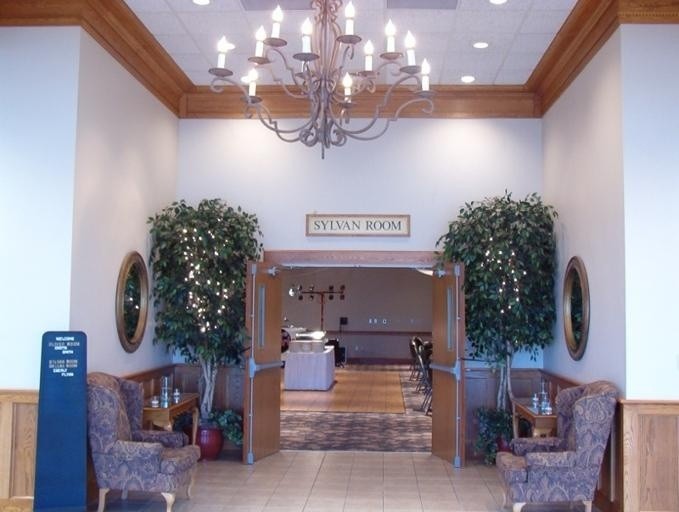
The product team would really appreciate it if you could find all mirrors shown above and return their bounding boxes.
[562,256,590,362]
[115,250,149,353]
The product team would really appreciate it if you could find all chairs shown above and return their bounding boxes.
[408,335,432,416]
[85,371,201,512]
[497,382,617,511]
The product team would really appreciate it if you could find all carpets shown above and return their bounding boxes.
[280,370,403,412]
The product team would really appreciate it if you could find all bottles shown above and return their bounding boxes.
[160,402,169,409]
[533,383,553,410]
[151,396,159,407]
[173,399,180,404]
[160,373,172,401]
[540,408,545,415]
[545,411,552,415]
[173,388,181,399]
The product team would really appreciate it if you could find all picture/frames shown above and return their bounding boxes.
[306,213,411,237]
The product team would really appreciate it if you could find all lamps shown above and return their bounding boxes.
[207,0,440,161]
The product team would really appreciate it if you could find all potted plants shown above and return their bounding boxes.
[442,194,558,461]
[148,198,260,463]
